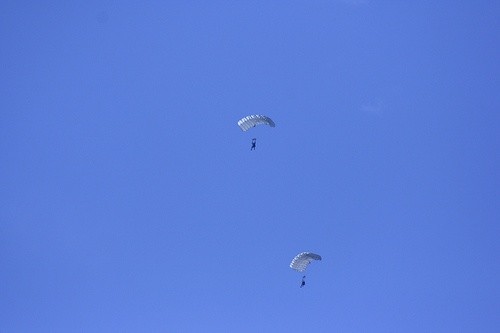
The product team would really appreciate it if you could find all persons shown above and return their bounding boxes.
[250,140,256,151]
[300,280,305,288]
